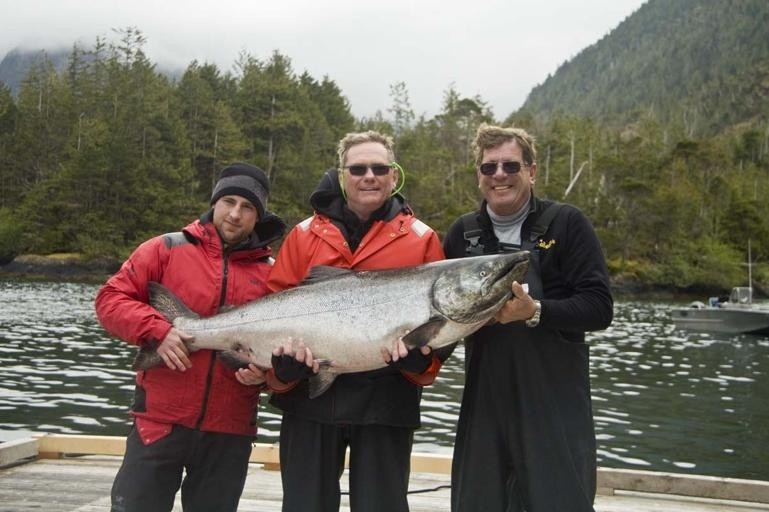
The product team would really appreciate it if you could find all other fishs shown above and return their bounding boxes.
[131,249,533,401]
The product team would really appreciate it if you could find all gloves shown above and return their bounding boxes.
[385,346,432,374]
[269,353,317,386]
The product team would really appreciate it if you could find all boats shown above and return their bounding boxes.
[653,286,768,338]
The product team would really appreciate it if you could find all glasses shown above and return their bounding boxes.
[340,165,391,175]
[479,162,531,174]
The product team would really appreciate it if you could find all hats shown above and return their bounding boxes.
[210,161,269,223]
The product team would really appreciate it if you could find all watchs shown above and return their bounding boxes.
[527,296,541,329]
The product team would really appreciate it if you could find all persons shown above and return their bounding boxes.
[437,122,613,511]
[264,128,448,512]
[94,161,289,512]
